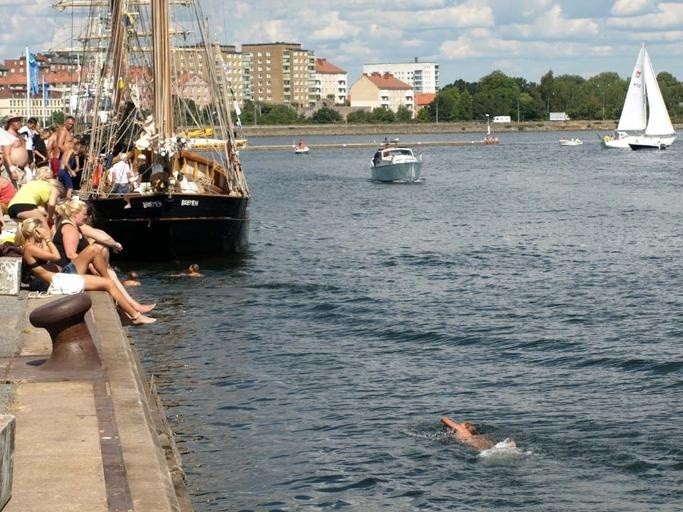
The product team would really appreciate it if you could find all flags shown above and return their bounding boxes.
[42,79,49,107]
[26,53,39,96]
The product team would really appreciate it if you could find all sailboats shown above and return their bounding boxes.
[72,2,250,258]
[600,41,677,148]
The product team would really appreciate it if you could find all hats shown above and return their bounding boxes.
[5,112,28,121]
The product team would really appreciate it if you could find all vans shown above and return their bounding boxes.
[491,116,511,122]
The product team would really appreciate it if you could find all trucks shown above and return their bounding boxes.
[550,112,571,120]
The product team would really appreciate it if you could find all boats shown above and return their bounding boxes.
[369,146,424,182]
[628,141,668,150]
[558,138,584,146]
[294,146,310,154]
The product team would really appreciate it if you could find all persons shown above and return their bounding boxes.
[438,415,519,458]
[382,143,391,158]
[485,135,490,145]
[297,143,303,150]
[0,111,163,327]
[490,136,496,145]
[178,262,205,278]
